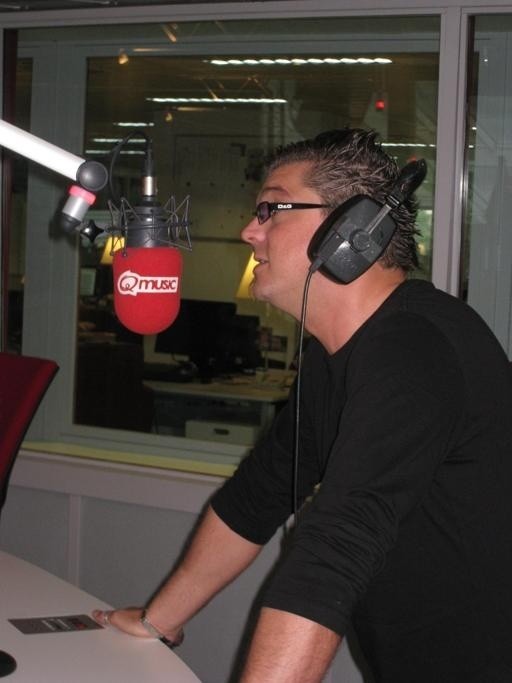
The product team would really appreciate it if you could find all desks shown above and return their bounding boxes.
[78,332,297,446]
[0,547,201,682]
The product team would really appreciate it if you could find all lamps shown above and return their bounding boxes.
[236,251,270,374]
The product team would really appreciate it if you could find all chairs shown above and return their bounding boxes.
[0,352,60,484]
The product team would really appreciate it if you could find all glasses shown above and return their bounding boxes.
[251,201,330,225]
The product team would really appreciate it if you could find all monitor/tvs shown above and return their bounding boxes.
[80,267,97,296]
[155,298,237,363]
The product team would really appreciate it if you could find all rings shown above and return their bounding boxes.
[104,611,111,626]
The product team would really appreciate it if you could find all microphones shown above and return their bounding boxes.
[110,147,184,336]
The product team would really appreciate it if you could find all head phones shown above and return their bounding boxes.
[307,158,429,286]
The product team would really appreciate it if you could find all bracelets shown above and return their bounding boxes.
[139,617,185,650]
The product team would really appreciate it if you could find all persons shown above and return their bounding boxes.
[91,129,511,683]
